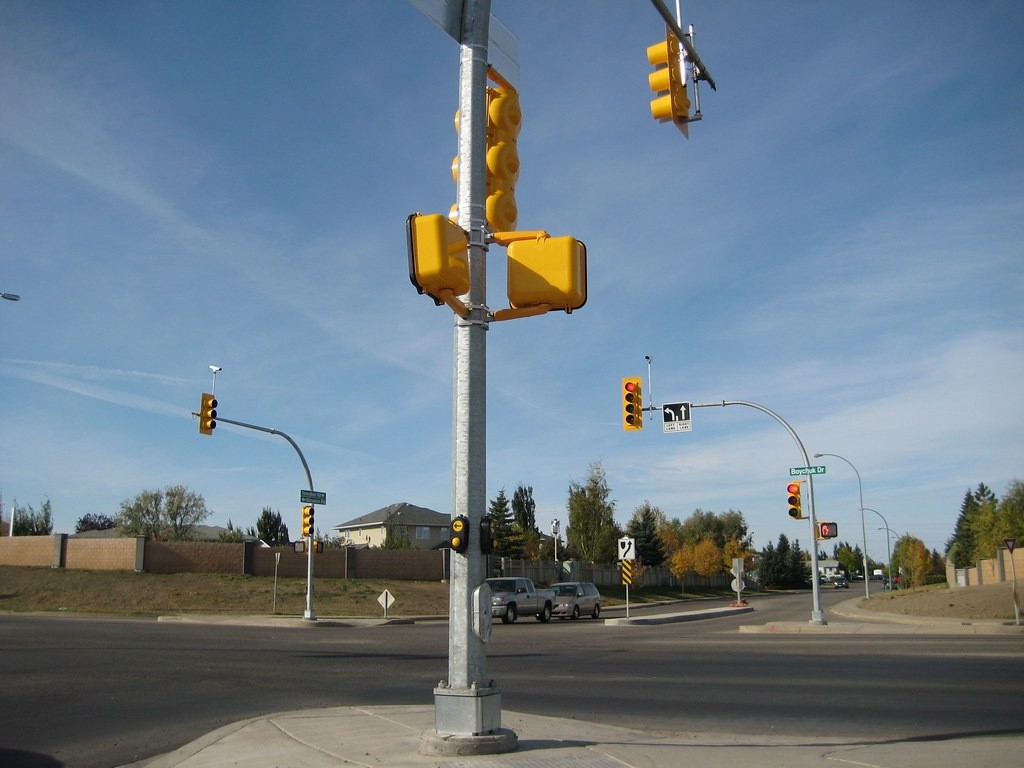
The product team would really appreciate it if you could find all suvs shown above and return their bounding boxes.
[535,581,601,621]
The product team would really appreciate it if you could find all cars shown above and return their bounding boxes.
[834,577,849,589]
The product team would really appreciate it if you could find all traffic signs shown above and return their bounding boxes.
[661,402,693,433]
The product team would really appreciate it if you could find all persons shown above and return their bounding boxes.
[882,578,887,591]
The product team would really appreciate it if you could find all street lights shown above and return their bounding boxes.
[813,453,870,600]
[878,528,902,556]
[858,508,893,592]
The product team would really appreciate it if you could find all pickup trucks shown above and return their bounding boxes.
[484,577,556,625]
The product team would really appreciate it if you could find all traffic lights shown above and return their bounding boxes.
[621,376,642,431]
[301,505,315,537]
[293,540,307,555]
[199,392,219,436]
[648,22,691,140]
[313,540,324,553]
[820,522,838,538]
[447,85,520,234]
[787,483,802,519]
